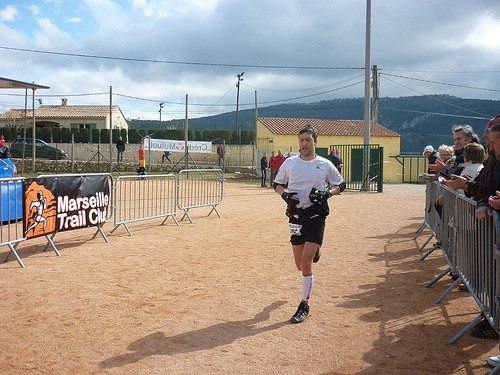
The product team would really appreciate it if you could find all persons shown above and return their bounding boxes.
[260,153,268,187]
[273,124,345,323]
[271,150,286,181]
[418,114,500,366]
[162,151,173,163]
[116,136,125,162]
[325,148,343,196]
[217,144,226,166]
[0,135,6,148]
[269,151,275,188]
[286,152,293,159]
[137,165,147,180]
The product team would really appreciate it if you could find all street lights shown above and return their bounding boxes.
[235,71,245,128]
[158,102,164,130]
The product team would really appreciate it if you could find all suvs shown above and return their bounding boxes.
[9,138,68,161]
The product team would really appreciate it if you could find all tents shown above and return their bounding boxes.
[0,78,49,173]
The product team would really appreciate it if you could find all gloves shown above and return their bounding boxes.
[309,189,333,204]
[282,192,300,207]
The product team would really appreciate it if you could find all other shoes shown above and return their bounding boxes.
[313,247,321,263]
[452,275,459,280]
[290,302,310,324]
[486,355,500,367]
[459,284,468,291]
[261,184,267,188]
[433,243,441,249]
[467,320,499,339]
[448,272,452,276]
